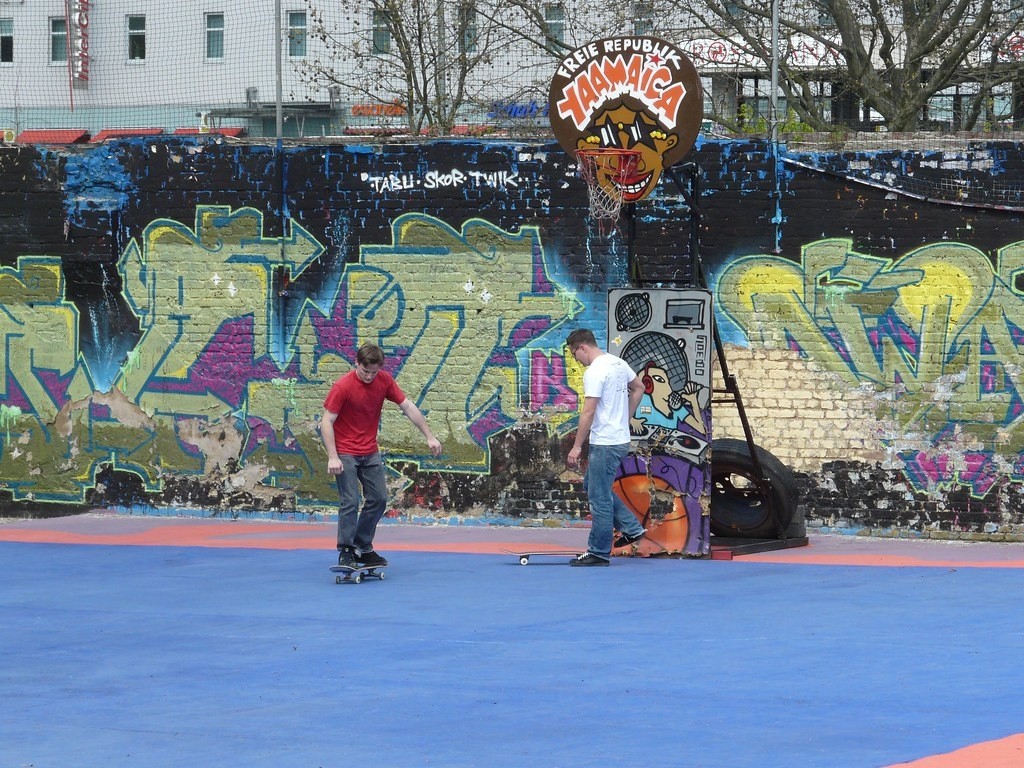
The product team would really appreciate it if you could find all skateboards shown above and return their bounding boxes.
[328,561,389,584]
[497,547,585,566]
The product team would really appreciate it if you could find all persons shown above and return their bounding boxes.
[321,344,442,568]
[567,328,646,566]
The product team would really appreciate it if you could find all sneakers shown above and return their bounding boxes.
[339,547,359,568]
[614,532,645,548]
[355,550,387,565]
[570,552,609,566]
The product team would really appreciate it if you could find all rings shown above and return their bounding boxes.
[571,462,575,463]
